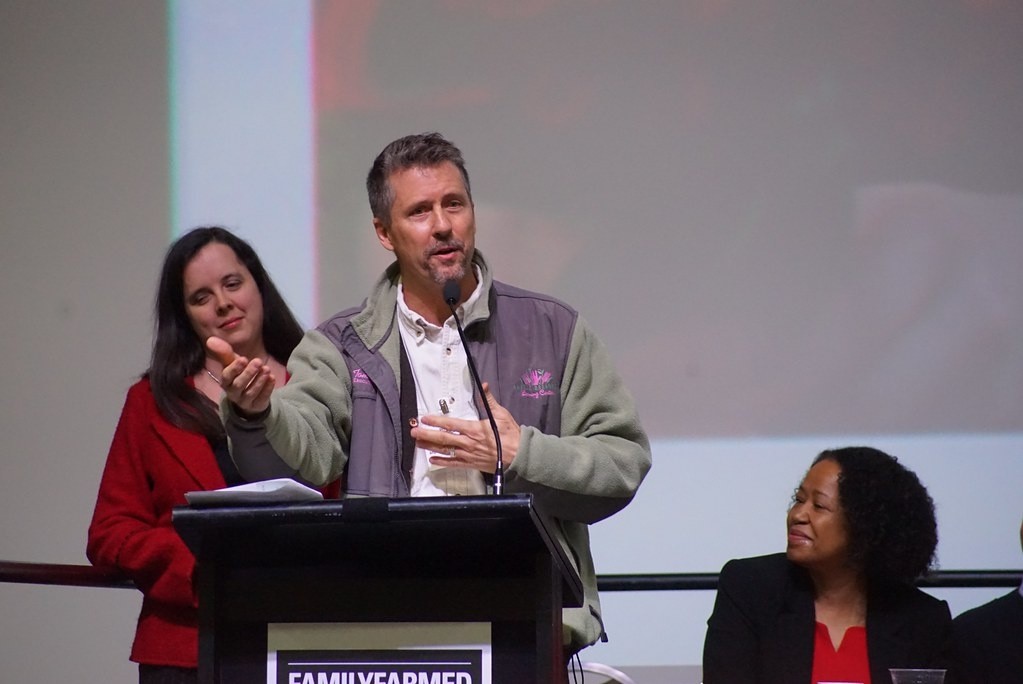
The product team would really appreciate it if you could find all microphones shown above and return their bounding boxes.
[442,278,505,495]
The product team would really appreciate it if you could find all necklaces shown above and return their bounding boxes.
[204,349,270,393]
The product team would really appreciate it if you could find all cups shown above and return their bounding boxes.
[889,668,946,684]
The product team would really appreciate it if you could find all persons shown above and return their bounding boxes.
[927,525,1023,684]
[211,128,654,684]
[703,447,962,684]
[85,225,344,684]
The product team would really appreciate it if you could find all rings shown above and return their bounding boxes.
[449,446,456,457]
